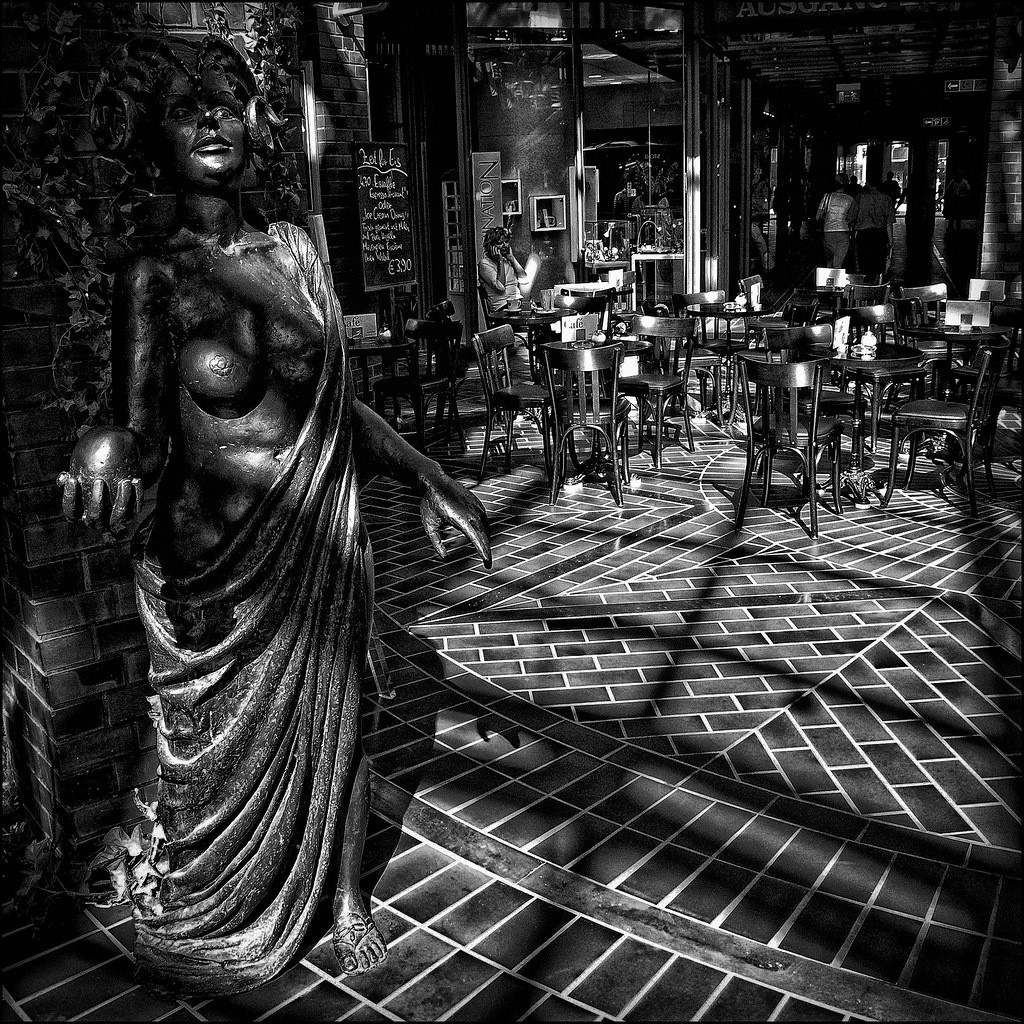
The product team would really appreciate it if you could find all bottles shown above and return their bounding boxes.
[861,331,876,345]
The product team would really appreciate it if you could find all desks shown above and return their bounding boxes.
[899,324,1012,455]
[488,308,579,384]
[808,344,925,453]
[685,302,775,397]
[541,340,652,439]
[348,339,423,441]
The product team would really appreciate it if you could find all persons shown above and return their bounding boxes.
[59,35,491,995]
[478,227,527,313]
[815,168,970,271]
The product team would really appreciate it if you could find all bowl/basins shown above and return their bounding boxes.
[850,344,877,356]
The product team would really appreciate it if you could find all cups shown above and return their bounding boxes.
[507,299,521,310]
[540,290,552,311]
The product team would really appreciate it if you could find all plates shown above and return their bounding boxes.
[535,308,559,315]
[851,352,876,358]
[503,308,522,311]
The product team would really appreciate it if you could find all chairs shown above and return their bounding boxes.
[346,269,1024,540]
